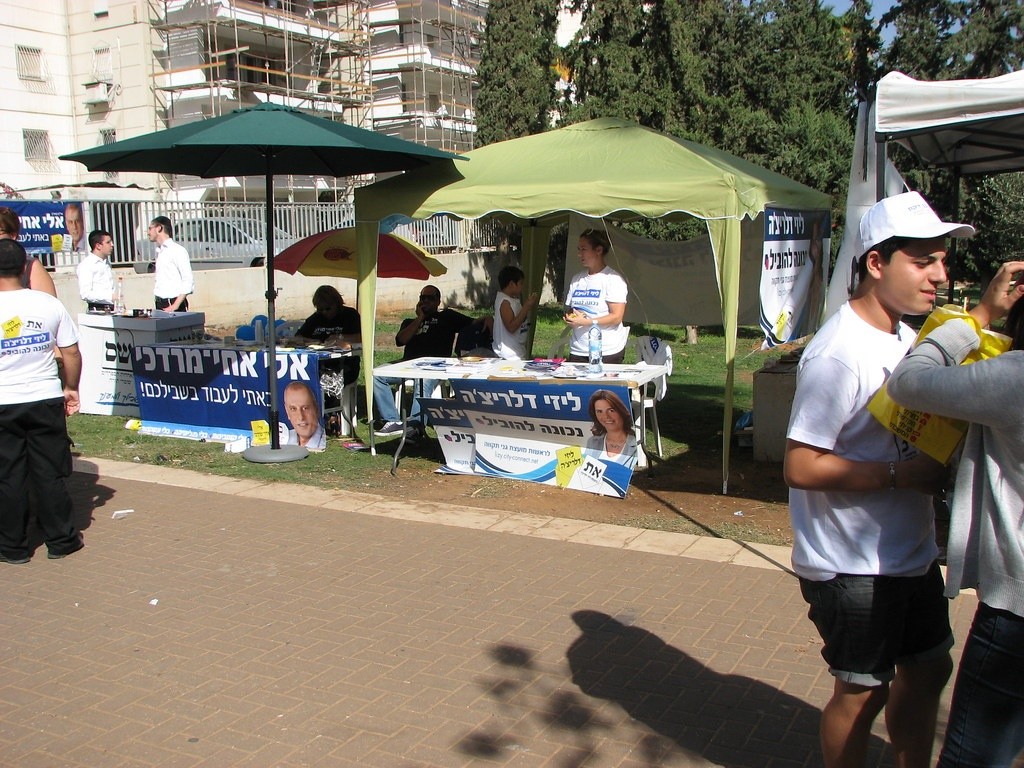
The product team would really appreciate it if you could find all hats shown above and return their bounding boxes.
[853,190,975,261]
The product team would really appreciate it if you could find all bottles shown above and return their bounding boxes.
[265,319,269,343]
[114,277,125,314]
[588,320,602,374]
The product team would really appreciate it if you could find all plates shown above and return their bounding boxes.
[237,341,257,346]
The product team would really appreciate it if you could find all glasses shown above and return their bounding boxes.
[419,295,436,301]
[317,304,335,312]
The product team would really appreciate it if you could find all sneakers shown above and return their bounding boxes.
[0,549,29,564]
[374,421,406,437]
[400,426,420,444]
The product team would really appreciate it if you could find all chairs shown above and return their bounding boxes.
[625,336,673,457]
[339,378,358,436]
[395,333,459,420]
[548,326,574,360]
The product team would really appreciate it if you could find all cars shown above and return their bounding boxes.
[329,217,450,253]
[131,217,301,271]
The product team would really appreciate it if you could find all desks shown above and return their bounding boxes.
[372,356,669,488]
[130,339,363,452]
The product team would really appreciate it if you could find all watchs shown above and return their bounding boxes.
[591,319,599,326]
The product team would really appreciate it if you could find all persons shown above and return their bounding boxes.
[584,389,638,471]
[784,191,978,768]
[563,228,630,364]
[0,238,82,564]
[65,205,86,252]
[284,381,326,453]
[147,216,194,312]
[373,285,461,436]
[283,285,362,437]
[886,261,1024,768]
[492,266,537,360]
[0,208,58,296]
[76,230,123,310]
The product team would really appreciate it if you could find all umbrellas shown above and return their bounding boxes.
[59,104,471,463]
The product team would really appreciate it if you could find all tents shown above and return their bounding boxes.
[829,67,1024,318]
[355,114,833,494]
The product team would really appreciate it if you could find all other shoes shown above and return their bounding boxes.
[46,534,85,559]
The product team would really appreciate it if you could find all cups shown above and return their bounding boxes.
[255,320,264,342]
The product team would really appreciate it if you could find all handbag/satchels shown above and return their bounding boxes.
[866,302,1013,472]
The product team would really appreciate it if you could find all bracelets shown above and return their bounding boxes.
[889,461,896,496]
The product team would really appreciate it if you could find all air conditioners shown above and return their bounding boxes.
[85,83,108,103]
